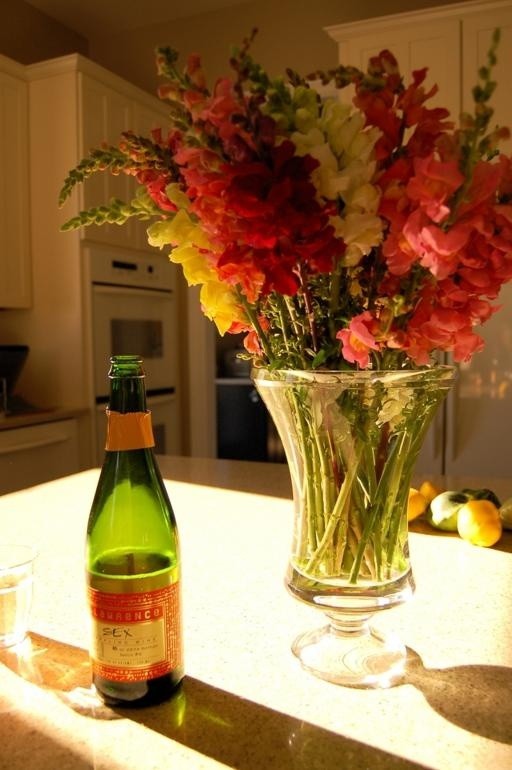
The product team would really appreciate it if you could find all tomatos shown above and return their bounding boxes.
[427,490,474,530]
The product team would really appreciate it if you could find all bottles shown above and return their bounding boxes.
[84,356,183,711]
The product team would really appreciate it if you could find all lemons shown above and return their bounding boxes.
[457,500,502,547]
[407,488,426,522]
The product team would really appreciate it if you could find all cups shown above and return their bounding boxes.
[0,561,36,642]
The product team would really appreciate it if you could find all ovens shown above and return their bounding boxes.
[79,244,187,475]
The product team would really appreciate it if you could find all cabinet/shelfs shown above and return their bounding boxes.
[324,1,512,165]
[29,51,182,255]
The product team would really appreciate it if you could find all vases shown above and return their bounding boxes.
[245,364,456,688]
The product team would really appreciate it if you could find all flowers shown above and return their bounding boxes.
[56,22,511,584]
